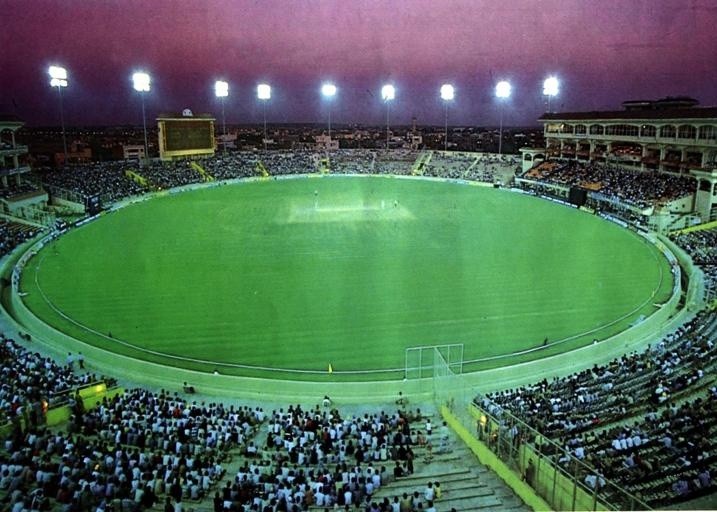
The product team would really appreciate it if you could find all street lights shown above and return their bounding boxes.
[214,80,230,155]
[439,83,455,151]
[380,85,396,149]
[494,80,511,153]
[320,84,337,138]
[542,75,561,113]
[131,71,151,166]
[47,65,70,164]
[257,84,271,153]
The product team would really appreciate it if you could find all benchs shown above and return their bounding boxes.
[476,216,716,510]
[2,317,509,512]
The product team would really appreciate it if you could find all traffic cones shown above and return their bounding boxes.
[326,361,334,373]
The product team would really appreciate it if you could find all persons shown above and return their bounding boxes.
[474,354,716,510]
[21,160,170,205]
[1,334,460,512]
[645,226,715,353]
[0,181,43,260]
[522,144,698,225]
[169,149,522,191]
